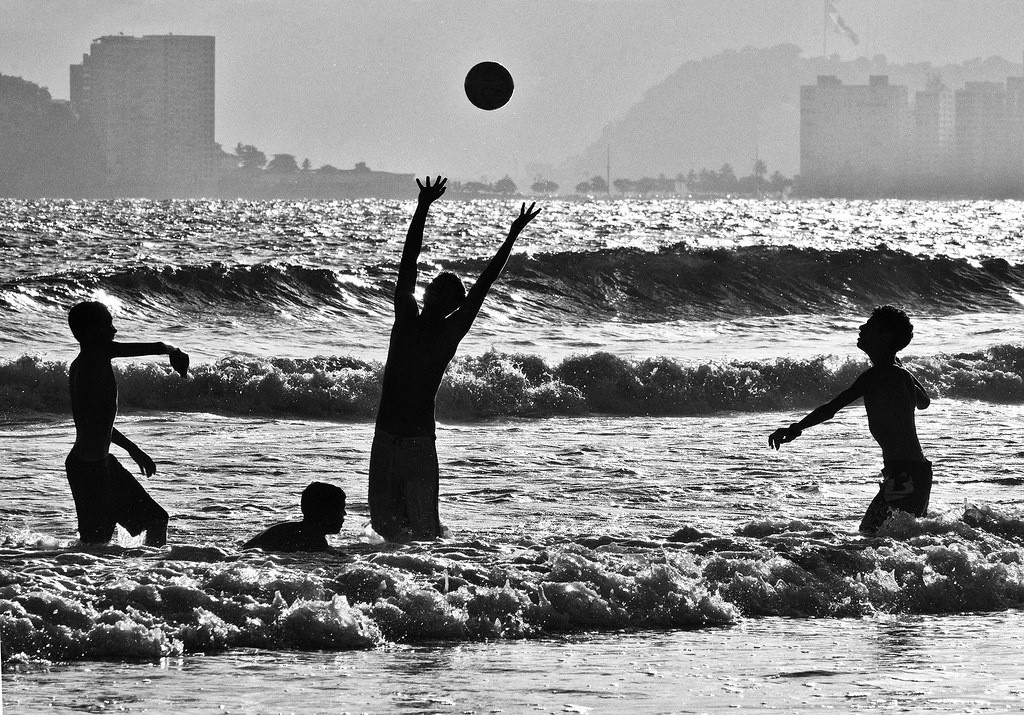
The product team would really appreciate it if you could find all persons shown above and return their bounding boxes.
[64,301,189,549]
[369,174,543,543]
[768,306,932,532]
[240,481,347,553]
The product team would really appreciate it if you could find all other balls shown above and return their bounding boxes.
[463,60,515,111]
